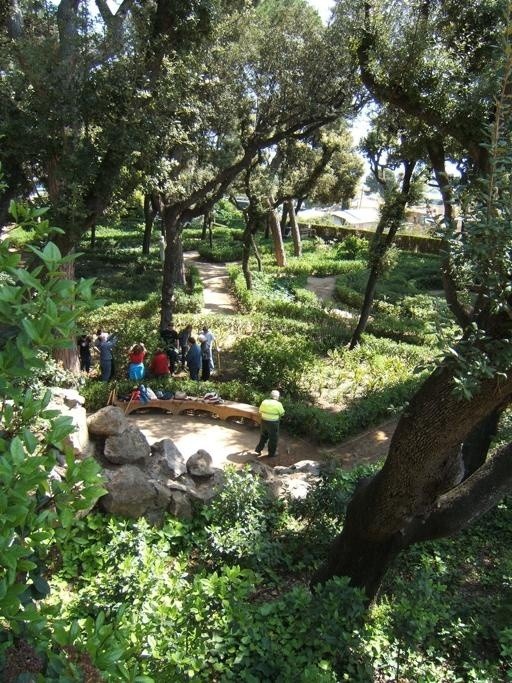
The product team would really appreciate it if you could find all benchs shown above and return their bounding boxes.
[109,391,262,426]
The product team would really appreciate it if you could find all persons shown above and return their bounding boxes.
[255,390,285,457]
[78,323,218,383]
